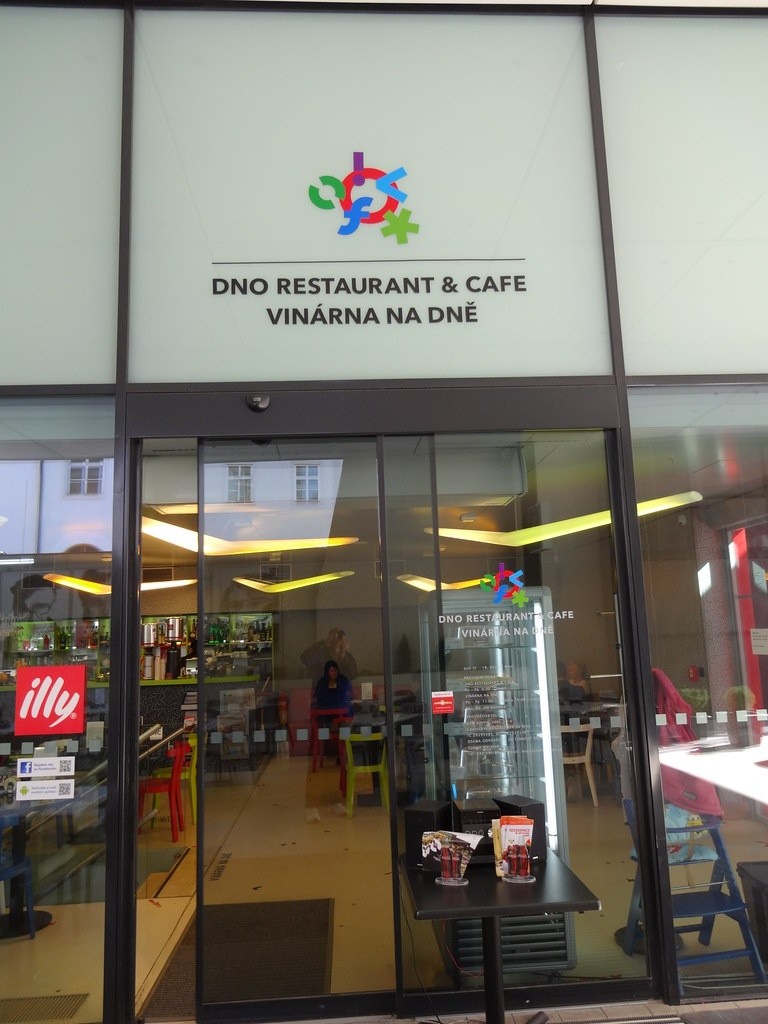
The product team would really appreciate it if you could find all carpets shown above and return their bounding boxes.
[135,898,336,1023]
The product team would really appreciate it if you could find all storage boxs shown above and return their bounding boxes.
[736,861,768,967]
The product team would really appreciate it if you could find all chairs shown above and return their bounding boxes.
[309,708,425,819]
[151,733,198,830]
[0,813,35,940]
[137,740,190,842]
[561,722,599,808]
[621,798,768,985]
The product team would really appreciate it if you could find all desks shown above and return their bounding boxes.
[340,722,421,807]
[399,852,601,1024]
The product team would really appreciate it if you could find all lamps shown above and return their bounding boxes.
[424,490,703,547]
[396,573,495,591]
[141,516,359,555]
[233,570,354,594]
[42,573,197,594]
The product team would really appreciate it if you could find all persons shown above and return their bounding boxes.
[173,640,198,680]
[557,659,592,800]
[313,659,353,766]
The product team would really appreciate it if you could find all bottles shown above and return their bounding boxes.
[30,628,54,650]
[61,625,107,650]
[208,619,273,644]
[190,619,199,640]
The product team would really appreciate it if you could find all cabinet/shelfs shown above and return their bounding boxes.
[0,722,185,910]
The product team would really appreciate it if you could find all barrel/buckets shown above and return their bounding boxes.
[166,617,183,641]
[142,622,158,647]
[142,655,154,680]
[165,649,180,679]
